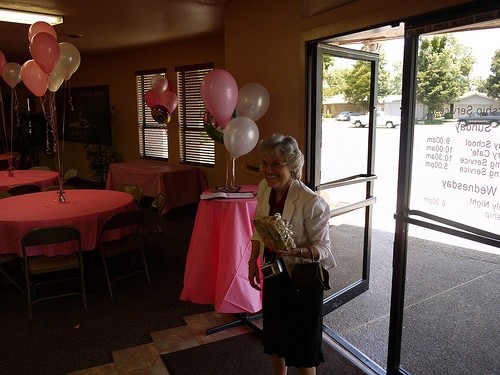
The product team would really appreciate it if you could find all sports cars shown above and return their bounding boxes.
[457,112,500,128]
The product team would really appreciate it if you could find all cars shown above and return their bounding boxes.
[336,111,353,121]
[349,111,401,128]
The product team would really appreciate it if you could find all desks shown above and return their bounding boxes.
[0,169,61,197]
[179,185,282,345]
[0,189,135,301]
[105,159,209,243]
[0,153,16,168]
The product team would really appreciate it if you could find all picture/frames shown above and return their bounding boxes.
[56,85,112,145]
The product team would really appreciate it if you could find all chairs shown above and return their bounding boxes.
[0,142,167,319]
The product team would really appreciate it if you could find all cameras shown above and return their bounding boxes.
[260,259,284,280]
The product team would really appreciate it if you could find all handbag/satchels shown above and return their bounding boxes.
[290,247,331,291]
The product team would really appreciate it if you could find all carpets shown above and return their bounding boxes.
[0,228,216,375]
[160,329,369,375]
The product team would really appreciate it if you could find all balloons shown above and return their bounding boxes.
[151,75,168,93]
[161,91,178,113]
[29,32,60,73]
[201,69,238,123]
[144,90,159,109]
[235,83,270,121]
[48,68,64,92]
[224,117,259,158]
[21,60,47,96]
[56,42,80,80]
[152,105,169,124]
[0,51,7,76]
[2,63,22,87]
[28,21,57,40]
[204,110,236,144]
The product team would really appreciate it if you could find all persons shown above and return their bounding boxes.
[249,134,337,375]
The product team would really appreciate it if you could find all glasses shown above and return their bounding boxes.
[262,161,287,169]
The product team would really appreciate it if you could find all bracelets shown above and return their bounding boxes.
[296,248,302,257]
